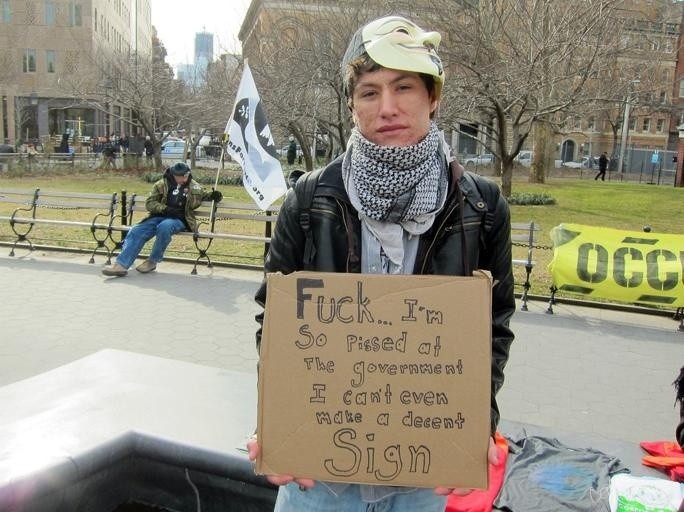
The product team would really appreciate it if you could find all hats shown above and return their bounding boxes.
[170,163,191,176]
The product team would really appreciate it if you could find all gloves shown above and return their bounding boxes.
[164,206,180,219]
[210,190,223,203]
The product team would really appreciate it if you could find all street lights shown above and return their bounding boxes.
[30,88,40,150]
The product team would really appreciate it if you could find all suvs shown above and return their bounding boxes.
[276,144,311,158]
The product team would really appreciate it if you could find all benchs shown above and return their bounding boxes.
[510,222,542,313]
[0,152,140,175]
[1,188,116,264]
[108,192,281,277]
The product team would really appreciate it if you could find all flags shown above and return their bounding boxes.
[224,64,289,212]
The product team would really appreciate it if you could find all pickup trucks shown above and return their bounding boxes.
[513,151,563,168]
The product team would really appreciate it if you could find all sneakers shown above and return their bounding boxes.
[136,260,156,273]
[104,263,127,276]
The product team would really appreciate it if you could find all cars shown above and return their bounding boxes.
[562,155,611,170]
[461,153,494,165]
[91,131,224,156]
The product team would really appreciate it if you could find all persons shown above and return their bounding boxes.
[119,136,129,153]
[144,136,153,160]
[595,152,608,180]
[247,16,517,511]
[102,164,224,276]
[286,137,296,165]
[0,139,14,171]
